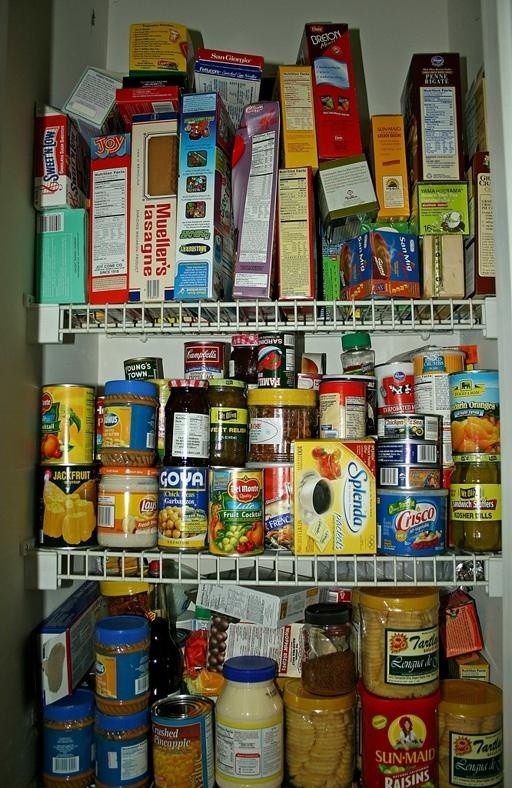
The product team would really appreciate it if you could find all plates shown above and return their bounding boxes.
[296,470,314,526]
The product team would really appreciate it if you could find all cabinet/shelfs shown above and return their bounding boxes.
[23,285,500,628]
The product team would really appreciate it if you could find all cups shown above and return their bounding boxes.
[302,476,335,517]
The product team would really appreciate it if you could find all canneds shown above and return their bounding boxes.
[36,586,506,788]
[34,331,501,559]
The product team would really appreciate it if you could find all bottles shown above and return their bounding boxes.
[147,561,183,703]
[339,332,376,376]
[213,655,285,787]
